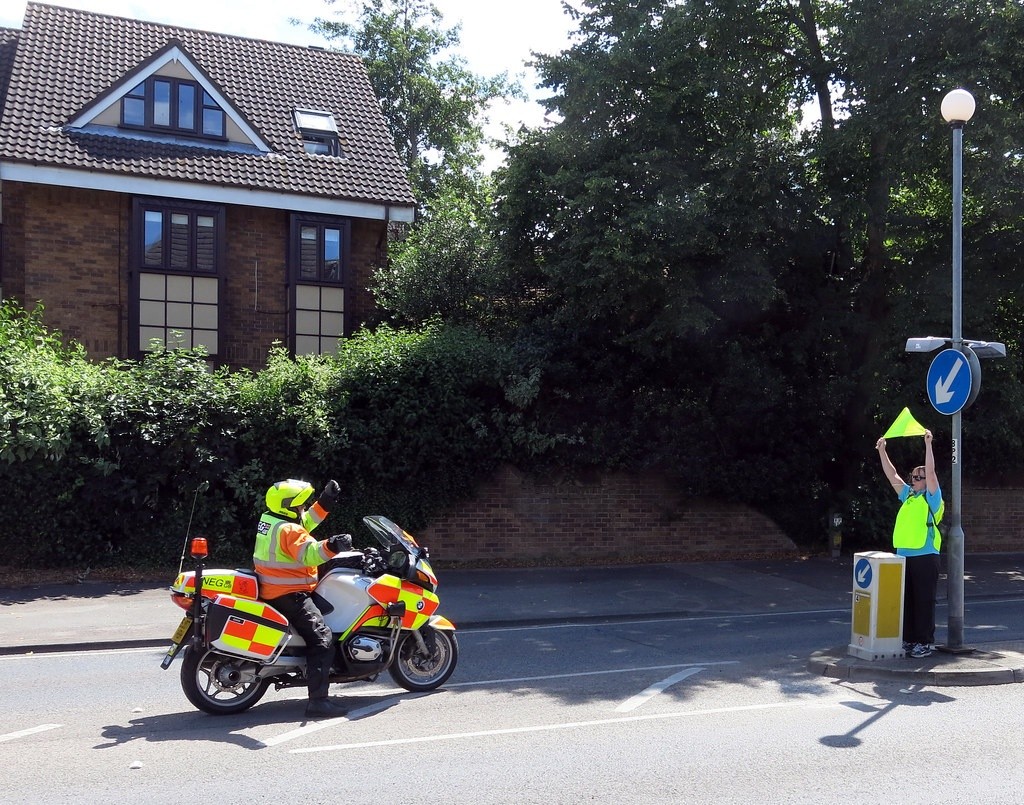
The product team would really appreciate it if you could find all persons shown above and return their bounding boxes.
[876,430,945,657]
[253,479,352,718]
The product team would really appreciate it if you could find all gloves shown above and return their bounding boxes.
[317,480,341,512]
[327,534,352,554]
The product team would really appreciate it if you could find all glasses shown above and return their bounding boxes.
[909,473,926,481]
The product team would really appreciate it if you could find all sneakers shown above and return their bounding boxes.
[902,642,932,658]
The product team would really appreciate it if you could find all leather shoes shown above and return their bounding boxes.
[305,699,350,718]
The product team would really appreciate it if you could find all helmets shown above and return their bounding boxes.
[265,479,316,523]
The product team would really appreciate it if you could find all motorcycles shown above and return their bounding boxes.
[160,480,459,716]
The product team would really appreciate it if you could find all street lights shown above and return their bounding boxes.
[904,88,1006,654]
